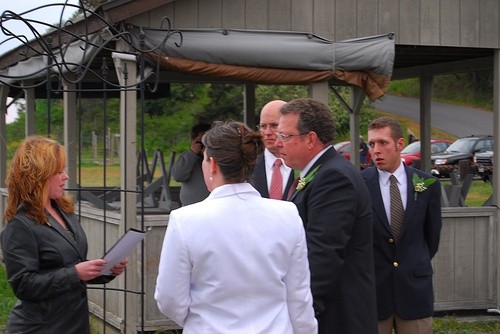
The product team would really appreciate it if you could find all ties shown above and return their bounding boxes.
[287,176,300,201]
[389,175,406,246]
[269,159,283,200]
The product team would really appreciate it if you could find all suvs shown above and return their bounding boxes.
[430,135,483,180]
[472,137,494,183]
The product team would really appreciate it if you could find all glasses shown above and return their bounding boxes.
[273,130,310,142]
[256,124,279,131]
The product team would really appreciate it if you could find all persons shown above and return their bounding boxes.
[0,136,129,334]
[173,101,443,334]
[154,122,318,334]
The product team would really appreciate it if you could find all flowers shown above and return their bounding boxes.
[289,164,321,201]
[413,172,437,201]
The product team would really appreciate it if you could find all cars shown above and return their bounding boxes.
[334,141,372,164]
[399,139,453,168]
